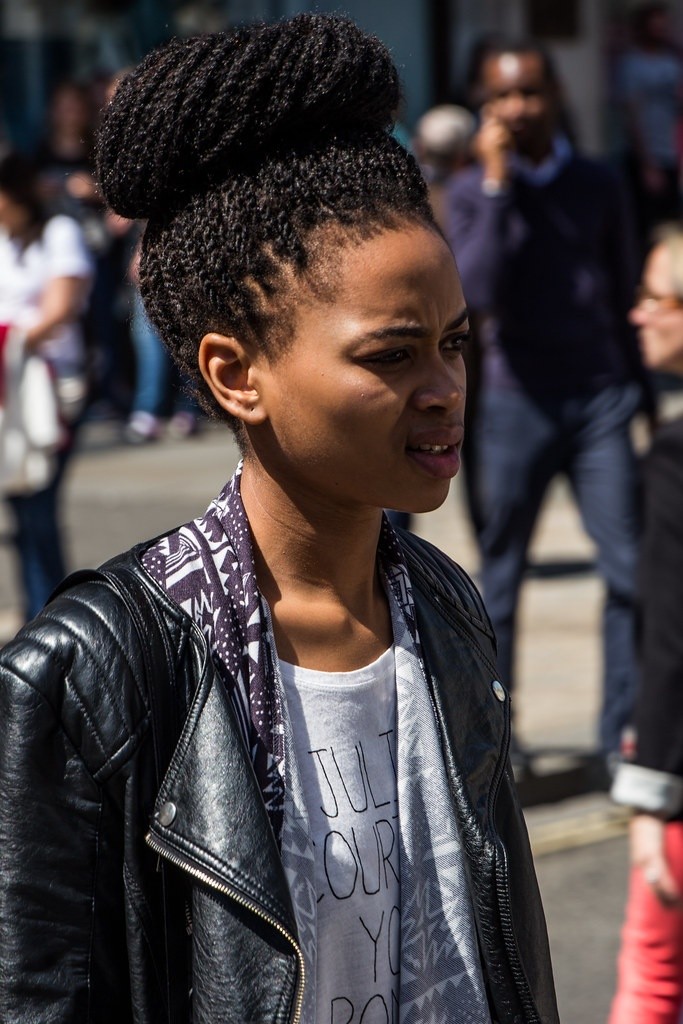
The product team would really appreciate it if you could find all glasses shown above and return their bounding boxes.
[636,291,683,319]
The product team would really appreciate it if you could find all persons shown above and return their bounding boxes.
[1,32,683,795]
[597,219,683,1024]
[0,15,565,1024]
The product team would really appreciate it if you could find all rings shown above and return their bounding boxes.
[646,870,659,883]
